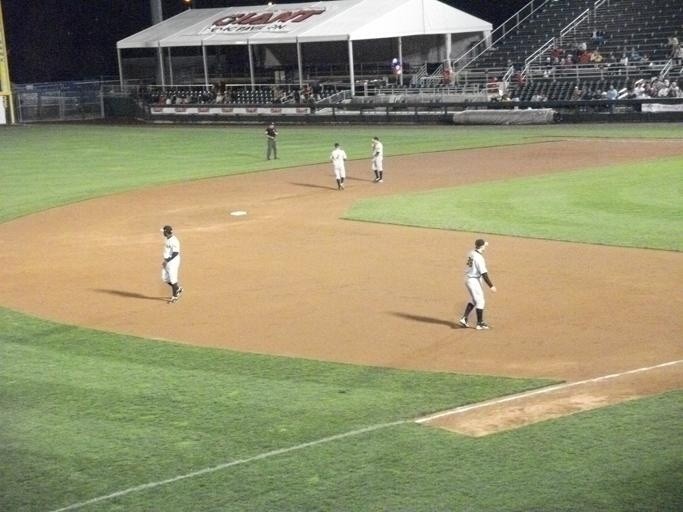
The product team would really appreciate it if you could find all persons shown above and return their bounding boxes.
[144,88,237,103]
[460,240,497,329]
[442,61,451,86]
[484,27,683,111]
[330,143,347,191]
[265,121,279,160]
[371,138,384,183]
[269,80,320,106]
[161,226,183,304]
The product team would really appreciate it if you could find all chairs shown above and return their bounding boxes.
[448,1,682,102]
[136,73,415,110]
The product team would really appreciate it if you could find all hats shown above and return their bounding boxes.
[160,226,172,232]
[475,239,489,246]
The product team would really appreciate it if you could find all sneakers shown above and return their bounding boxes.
[460,317,469,327]
[338,183,344,191]
[374,177,384,183]
[476,322,491,330]
[168,287,184,304]
